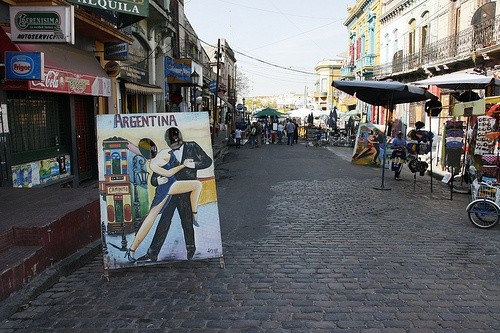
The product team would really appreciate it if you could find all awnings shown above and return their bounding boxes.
[0,26,113,97]
[121,77,163,95]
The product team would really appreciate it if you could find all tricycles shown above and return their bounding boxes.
[466,178,500,228]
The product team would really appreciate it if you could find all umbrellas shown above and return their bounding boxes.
[254,108,285,117]
[331,72,500,188]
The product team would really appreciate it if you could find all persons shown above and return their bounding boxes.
[235,125,242,148]
[391,131,407,180]
[250,122,257,149]
[276,119,299,146]
